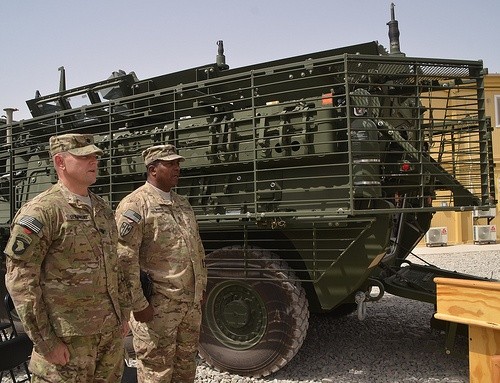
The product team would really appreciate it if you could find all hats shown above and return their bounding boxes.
[142,144,185,165]
[49,133,104,156]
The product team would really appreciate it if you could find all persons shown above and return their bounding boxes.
[115,144,209,383]
[3,133,132,383]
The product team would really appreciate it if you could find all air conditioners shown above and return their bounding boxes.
[474,198,496,218]
[425,227,448,248]
[474,225,497,245]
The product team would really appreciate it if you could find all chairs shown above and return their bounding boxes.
[0,292,34,383]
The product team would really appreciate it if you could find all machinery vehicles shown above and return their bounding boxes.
[0,0,500,379]
